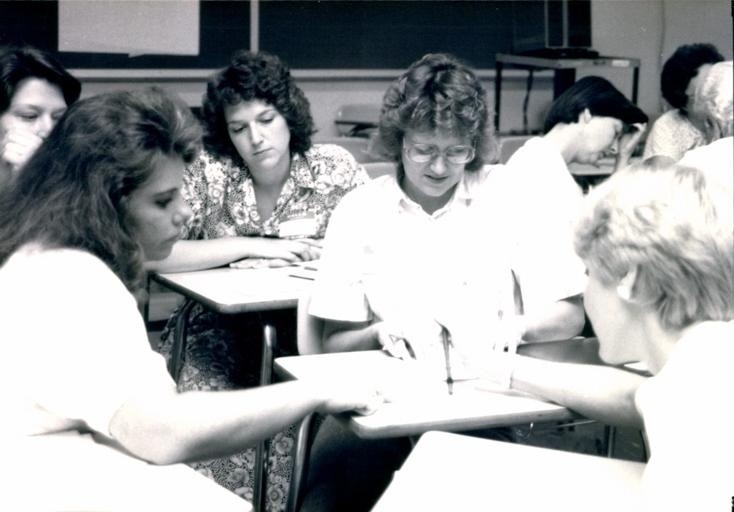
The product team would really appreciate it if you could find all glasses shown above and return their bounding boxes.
[402,143,477,164]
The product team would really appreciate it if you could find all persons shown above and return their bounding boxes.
[2,45,82,179]
[2,89,396,511]
[137,48,371,269]
[312,43,732,511]
